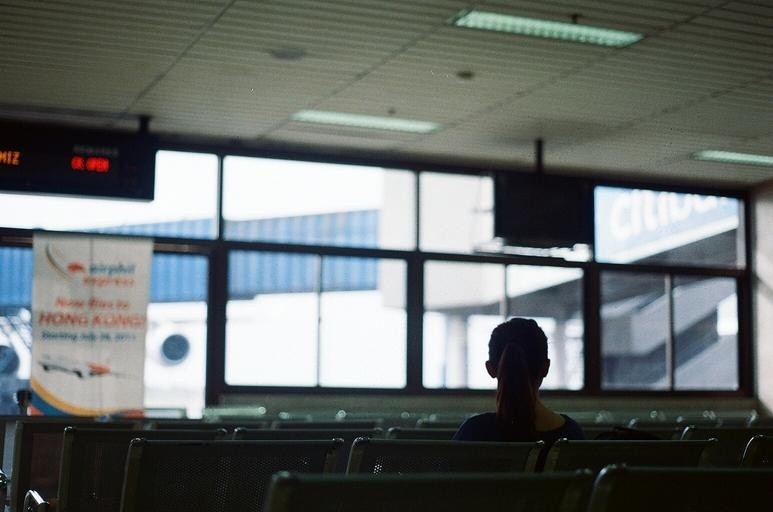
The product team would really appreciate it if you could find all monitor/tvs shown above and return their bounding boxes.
[494,168,594,243]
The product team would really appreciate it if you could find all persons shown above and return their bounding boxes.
[451,318,582,472]
[16,391,33,416]
